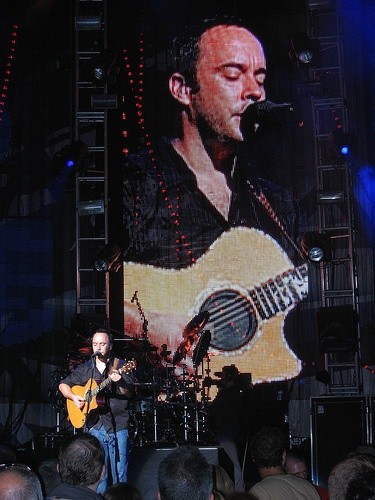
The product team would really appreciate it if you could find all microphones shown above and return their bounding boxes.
[131,291,138,303]
[91,351,100,357]
[253,100,291,119]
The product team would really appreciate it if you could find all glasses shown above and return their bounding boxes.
[0,462,34,476]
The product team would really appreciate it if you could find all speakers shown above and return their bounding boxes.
[317,304,358,352]
[71,312,108,337]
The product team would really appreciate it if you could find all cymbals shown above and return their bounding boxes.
[171,310,211,367]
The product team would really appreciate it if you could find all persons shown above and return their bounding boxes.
[207,365,245,492]
[59,328,132,497]
[108,16,314,381]
[0,433,375,500]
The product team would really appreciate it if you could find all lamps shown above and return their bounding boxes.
[288,32,313,71]
[299,232,333,263]
[94,243,122,273]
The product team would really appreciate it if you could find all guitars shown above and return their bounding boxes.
[66,356,136,428]
[124,225,311,385]
